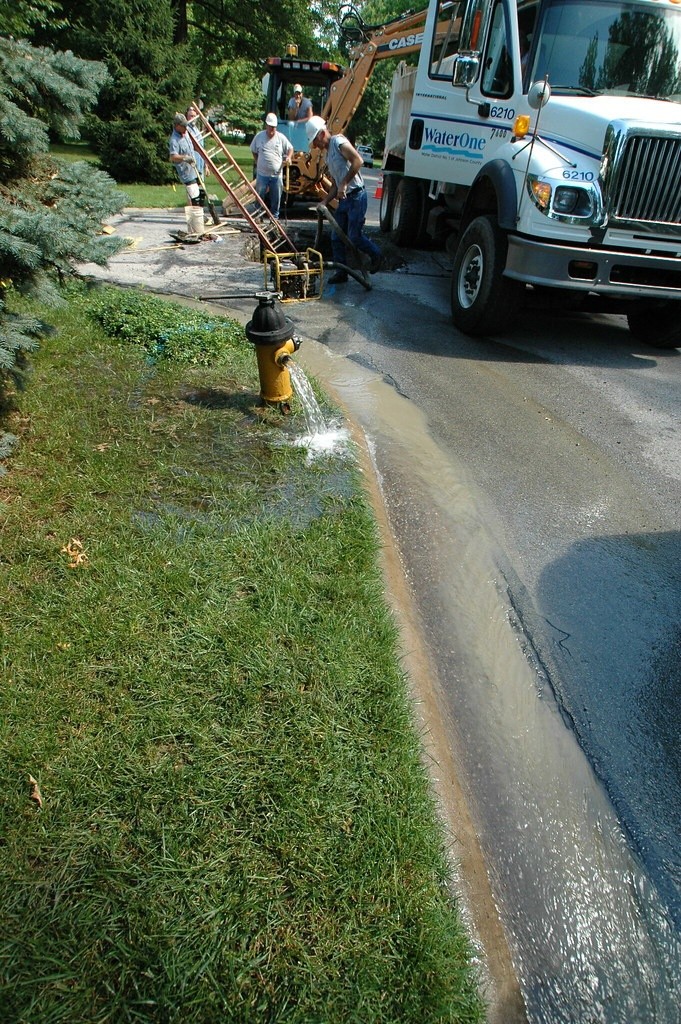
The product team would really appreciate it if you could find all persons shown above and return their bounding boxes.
[249,112,293,226]
[169,112,201,205]
[305,115,383,284]
[184,106,213,226]
[505,29,545,92]
[287,83,314,123]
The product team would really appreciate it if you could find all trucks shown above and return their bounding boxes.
[281,1,681,348]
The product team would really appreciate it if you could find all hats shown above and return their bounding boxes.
[293,84,303,94]
[174,114,191,127]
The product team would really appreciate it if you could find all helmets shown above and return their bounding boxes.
[265,113,279,128]
[305,115,326,145]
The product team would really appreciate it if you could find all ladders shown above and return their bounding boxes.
[175,100,300,261]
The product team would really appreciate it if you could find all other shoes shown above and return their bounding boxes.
[327,269,349,284]
[369,257,387,274]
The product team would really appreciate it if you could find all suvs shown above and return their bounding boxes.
[355,145,374,168]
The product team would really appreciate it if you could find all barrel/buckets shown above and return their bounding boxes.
[184,205,204,233]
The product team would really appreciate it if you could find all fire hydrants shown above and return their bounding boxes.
[245,291,304,415]
